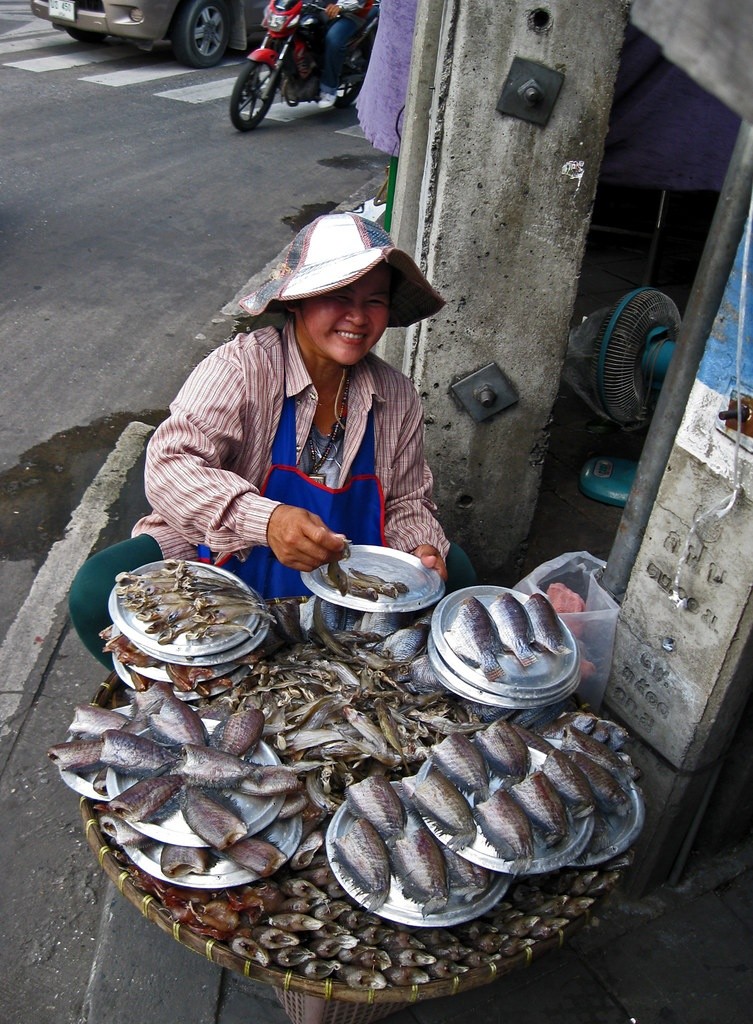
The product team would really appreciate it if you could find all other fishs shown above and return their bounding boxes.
[50,549,643,990]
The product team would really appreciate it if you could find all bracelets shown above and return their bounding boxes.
[339,6,342,9]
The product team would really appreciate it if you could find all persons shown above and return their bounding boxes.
[69,212,474,670]
[318,0,374,109]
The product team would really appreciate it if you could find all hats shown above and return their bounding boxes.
[237,213,443,328]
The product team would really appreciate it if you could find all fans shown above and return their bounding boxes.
[579,287,681,508]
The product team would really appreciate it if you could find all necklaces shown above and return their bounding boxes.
[308,378,349,485]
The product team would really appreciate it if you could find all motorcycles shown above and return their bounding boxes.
[228,0,380,132]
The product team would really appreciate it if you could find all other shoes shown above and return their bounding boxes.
[318,90,338,109]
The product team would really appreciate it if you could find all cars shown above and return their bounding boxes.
[31,0,273,70]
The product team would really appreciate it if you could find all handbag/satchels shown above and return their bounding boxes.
[511,550,620,709]
